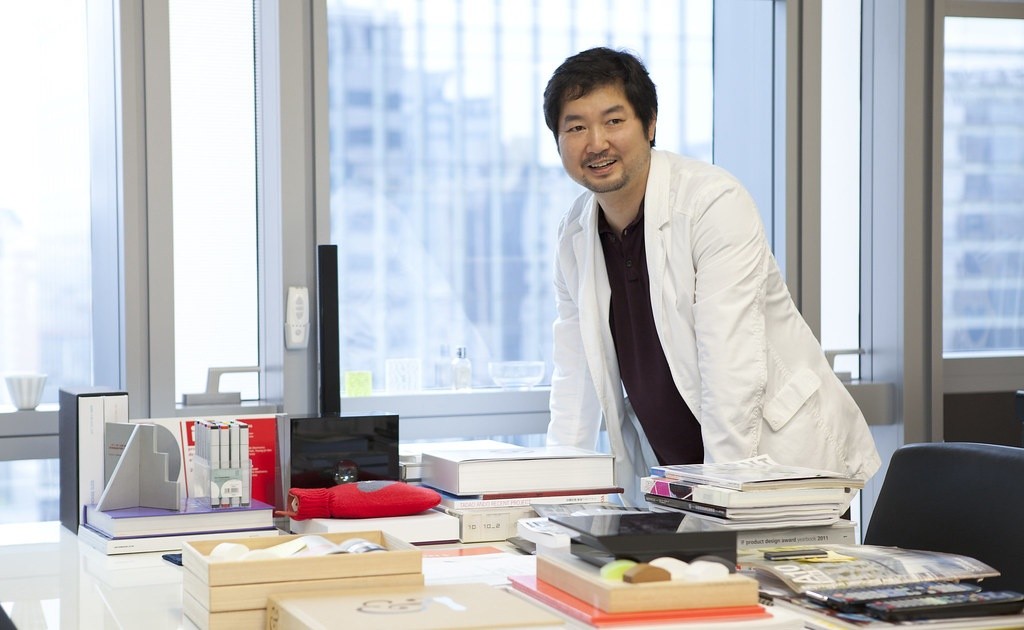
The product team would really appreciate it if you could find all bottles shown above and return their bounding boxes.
[450,346,471,391]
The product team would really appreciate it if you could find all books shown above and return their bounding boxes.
[396,435,1024,630]
[55,383,277,556]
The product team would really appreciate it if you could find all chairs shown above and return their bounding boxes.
[865,441,1024,598]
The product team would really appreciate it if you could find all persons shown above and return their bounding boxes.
[544,47,885,519]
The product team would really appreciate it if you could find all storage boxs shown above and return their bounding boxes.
[181,529,565,630]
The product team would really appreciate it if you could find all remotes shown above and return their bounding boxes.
[806,579,1024,623]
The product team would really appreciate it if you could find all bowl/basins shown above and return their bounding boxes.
[488,361,546,387]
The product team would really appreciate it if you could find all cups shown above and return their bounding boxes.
[385,358,422,392]
[4,373,48,410]
[345,370,372,396]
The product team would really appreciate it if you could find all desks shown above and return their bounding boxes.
[0,520,1024,630]
